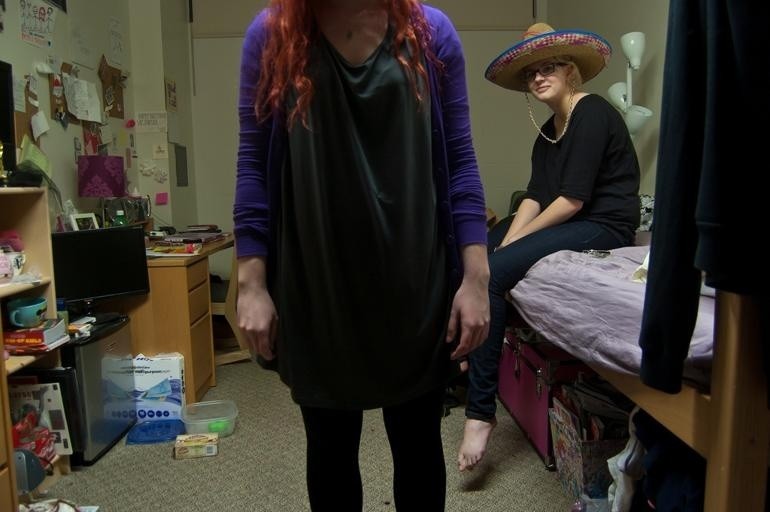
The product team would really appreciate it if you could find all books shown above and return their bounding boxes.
[5,335,70,353]
[152,242,203,256]
[4,317,67,344]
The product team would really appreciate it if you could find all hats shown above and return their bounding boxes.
[484,22,613,93]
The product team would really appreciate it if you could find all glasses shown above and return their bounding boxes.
[521,61,568,83]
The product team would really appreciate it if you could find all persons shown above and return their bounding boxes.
[222,1,493,511]
[439,22,641,473]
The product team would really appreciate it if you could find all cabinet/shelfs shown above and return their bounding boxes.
[1,186,71,511]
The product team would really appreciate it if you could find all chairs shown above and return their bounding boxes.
[209,238,251,364]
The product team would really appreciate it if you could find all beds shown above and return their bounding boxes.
[493,227,770,511]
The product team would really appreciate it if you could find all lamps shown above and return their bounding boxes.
[607,31,653,136]
[77,155,126,228]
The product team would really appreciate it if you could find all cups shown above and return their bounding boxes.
[8,251,26,278]
[10,296,48,330]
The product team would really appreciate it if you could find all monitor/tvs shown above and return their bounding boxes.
[51,223,150,325]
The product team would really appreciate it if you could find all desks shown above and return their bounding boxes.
[94,229,234,407]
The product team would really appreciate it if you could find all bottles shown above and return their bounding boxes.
[112,209,130,227]
